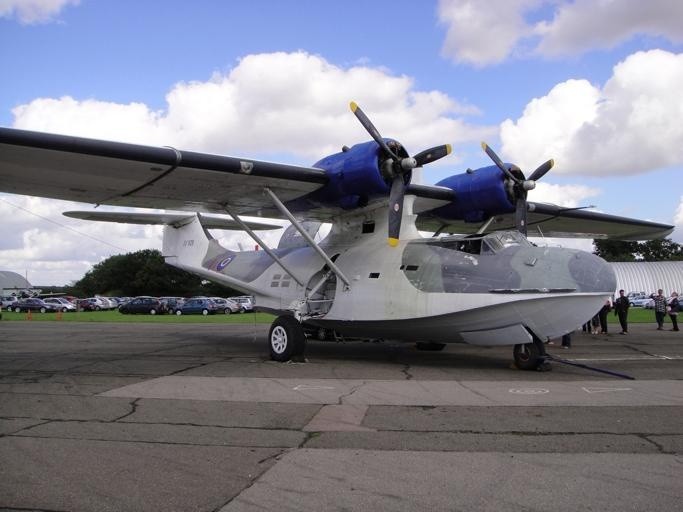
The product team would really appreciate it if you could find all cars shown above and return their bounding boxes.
[627,292,683,311]
[0,288,253,315]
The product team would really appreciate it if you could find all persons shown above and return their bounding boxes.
[591,313,600,335]
[614,289,630,336]
[665,291,681,332]
[582,320,591,334]
[596,298,611,335]
[560,334,571,349]
[649,288,668,330]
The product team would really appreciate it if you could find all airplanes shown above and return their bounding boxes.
[0,101,675,371]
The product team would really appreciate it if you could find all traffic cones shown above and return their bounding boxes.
[26,310,31,320]
[56,309,62,320]
